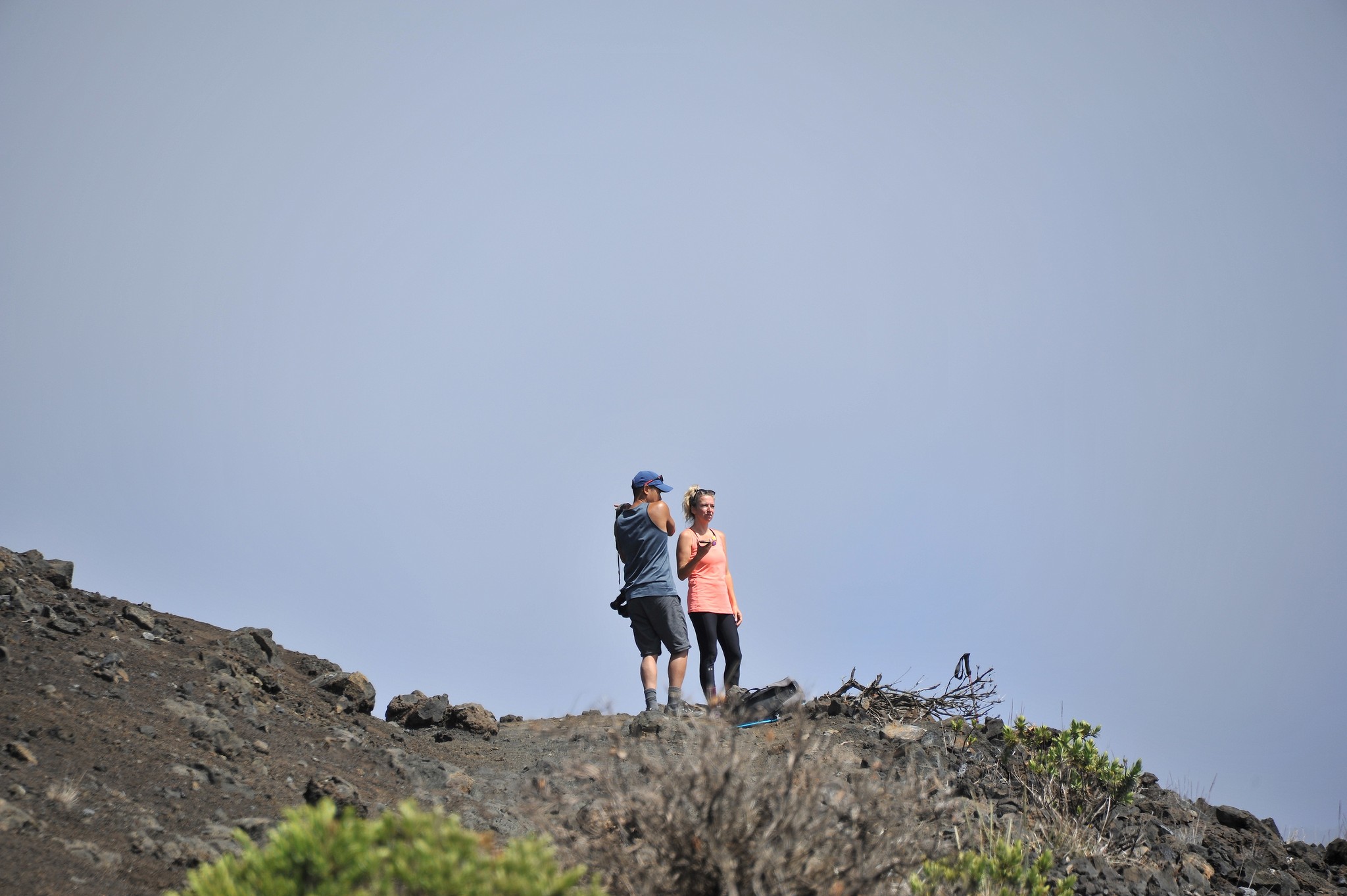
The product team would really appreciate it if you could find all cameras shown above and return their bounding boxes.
[610,588,627,610]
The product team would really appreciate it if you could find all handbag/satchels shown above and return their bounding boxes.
[727,676,800,725]
[610,588,629,618]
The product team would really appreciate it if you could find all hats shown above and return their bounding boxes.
[631,471,673,493]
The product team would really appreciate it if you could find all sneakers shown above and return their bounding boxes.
[645,708,659,713]
[663,699,705,719]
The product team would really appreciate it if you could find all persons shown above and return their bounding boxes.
[676,485,743,718]
[610,471,706,717]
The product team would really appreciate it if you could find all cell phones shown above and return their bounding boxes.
[699,539,711,543]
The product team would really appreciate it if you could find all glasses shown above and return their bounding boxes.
[693,489,715,505]
[644,475,663,486]
